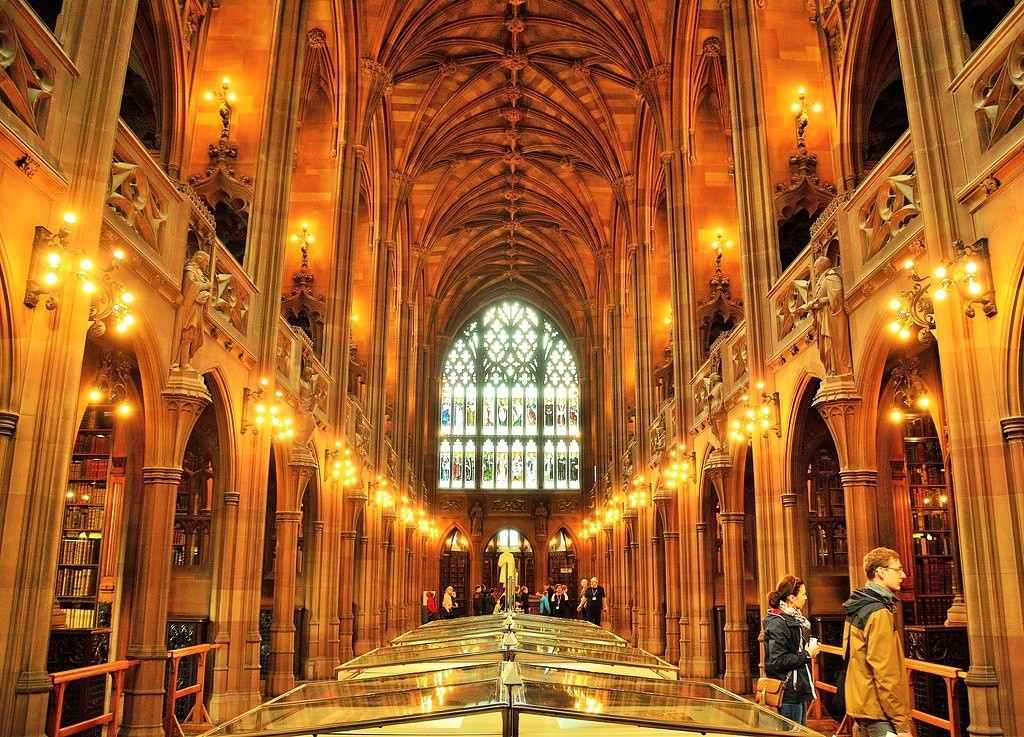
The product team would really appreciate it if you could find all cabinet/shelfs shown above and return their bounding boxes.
[440,551,578,619]
[712,413,972,737]
[50,402,303,737]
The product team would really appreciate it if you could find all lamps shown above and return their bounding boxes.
[934,238,997,321]
[577,497,624,540]
[367,476,439,538]
[889,260,936,345]
[890,349,928,422]
[204,78,236,140]
[628,477,652,509]
[241,378,294,442]
[23,211,96,311]
[324,441,357,485]
[730,382,782,446]
[86,340,135,415]
[89,248,134,338]
[290,221,314,272]
[792,88,821,147]
[665,445,697,489]
[712,229,733,272]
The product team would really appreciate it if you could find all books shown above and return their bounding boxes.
[171,479,189,567]
[807,449,848,566]
[903,417,956,627]
[53,411,115,629]
[296,511,304,573]
[715,501,723,573]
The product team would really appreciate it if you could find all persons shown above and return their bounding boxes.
[792,256,853,377]
[498,546,517,587]
[579,579,588,620]
[654,427,666,488]
[425,586,458,622]
[423,495,428,511]
[762,575,820,732]
[842,548,912,737]
[577,577,608,626]
[354,424,367,483]
[604,481,612,503]
[473,584,531,616]
[622,459,633,507]
[535,502,548,535]
[471,502,483,535]
[704,373,729,453]
[588,496,595,521]
[536,577,569,619]
[408,479,416,500]
[293,367,317,451]
[169,251,212,372]
[387,458,395,486]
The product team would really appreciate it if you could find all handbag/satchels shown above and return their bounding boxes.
[755,677,786,707]
[827,667,846,724]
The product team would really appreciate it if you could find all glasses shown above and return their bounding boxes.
[876,566,903,574]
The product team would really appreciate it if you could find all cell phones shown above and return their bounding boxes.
[808,634,819,648]
